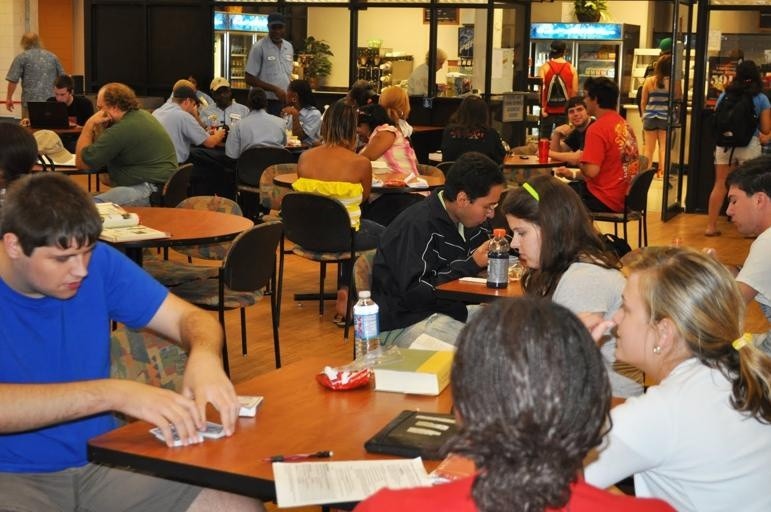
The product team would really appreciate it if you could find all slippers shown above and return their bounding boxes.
[332,289,347,327]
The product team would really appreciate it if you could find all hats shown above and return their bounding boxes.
[209,76,230,90]
[172,80,203,102]
[267,13,285,27]
[659,37,671,51]
[32,129,72,163]
[548,40,565,58]
[733,60,756,74]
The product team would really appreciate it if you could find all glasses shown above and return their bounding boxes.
[470,200,498,212]
[356,109,370,117]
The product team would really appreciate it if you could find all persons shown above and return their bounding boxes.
[1,10,770,511]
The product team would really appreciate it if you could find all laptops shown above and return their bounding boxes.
[26,101,76,129]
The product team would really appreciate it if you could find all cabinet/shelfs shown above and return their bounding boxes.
[626,47,721,105]
[355,53,417,97]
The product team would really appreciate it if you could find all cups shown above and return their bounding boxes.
[538,138,551,164]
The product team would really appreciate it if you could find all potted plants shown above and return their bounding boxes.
[571,2,617,26]
[295,36,335,89]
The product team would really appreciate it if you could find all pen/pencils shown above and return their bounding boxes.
[260,451,333,461]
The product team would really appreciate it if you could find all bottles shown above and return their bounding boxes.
[486,229,511,288]
[230,36,253,90]
[353,291,381,361]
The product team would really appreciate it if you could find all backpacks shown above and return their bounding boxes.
[546,61,568,105]
[712,91,755,166]
